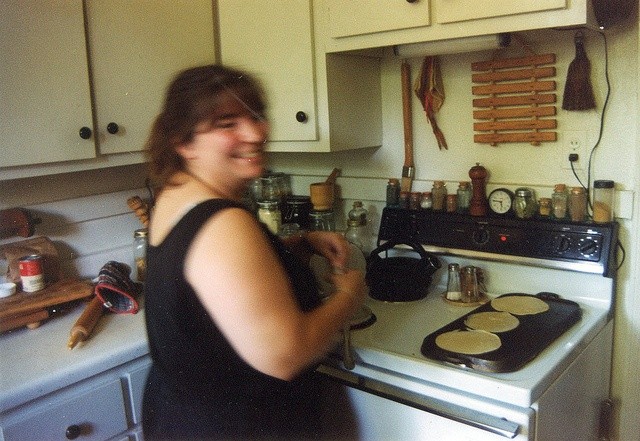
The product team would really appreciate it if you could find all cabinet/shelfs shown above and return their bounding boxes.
[320,0,605,56]
[1,1,216,180]
[0,378,131,441]
[126,363,153,440]
[215,1,384,154]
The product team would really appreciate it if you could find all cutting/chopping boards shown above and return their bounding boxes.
[0,275,94,324]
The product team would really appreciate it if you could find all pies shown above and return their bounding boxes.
[491,295,549,315]
[435,329,501,354]
[465,312,521,333]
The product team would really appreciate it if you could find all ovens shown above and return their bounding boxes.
[322,355,535,441]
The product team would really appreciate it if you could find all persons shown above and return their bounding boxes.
[140,65,368,440]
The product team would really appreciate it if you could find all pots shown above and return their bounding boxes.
[321,295,373,372]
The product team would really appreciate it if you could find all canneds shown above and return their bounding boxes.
[443,194,457,213]
[18,255,46,294]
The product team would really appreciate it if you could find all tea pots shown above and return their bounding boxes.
[364,235,443,301]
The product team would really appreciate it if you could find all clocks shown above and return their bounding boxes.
[487,187,514,214]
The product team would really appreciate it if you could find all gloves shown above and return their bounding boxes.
[95,260,143,314]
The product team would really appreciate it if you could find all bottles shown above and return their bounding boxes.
[512,186,538,220]
[459,265,481,303]
[568,185,588,222]
[133,229,148,282]
[249,178,265,202]
[255,201,282,237]
[398,191,410,209]
[348,201,366,221]
[386,179,401,207]
[457,182,473,211]
[420,192,433,210]
[308,210,335,233]
[592,178,615,225]
[431,181,447,211]
[445,195,458,214]
[551,183,569,219]
[283,196,313,230]
[409,192,421,210]
[260,173,281,202]
[344,219,373,259]
[278,173,292,197]
[538,196,553,217]
[445,261,463,302]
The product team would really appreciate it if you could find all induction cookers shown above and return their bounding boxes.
[321,247,614,410]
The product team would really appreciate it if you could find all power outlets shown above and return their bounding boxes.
[562,130,588,170]
